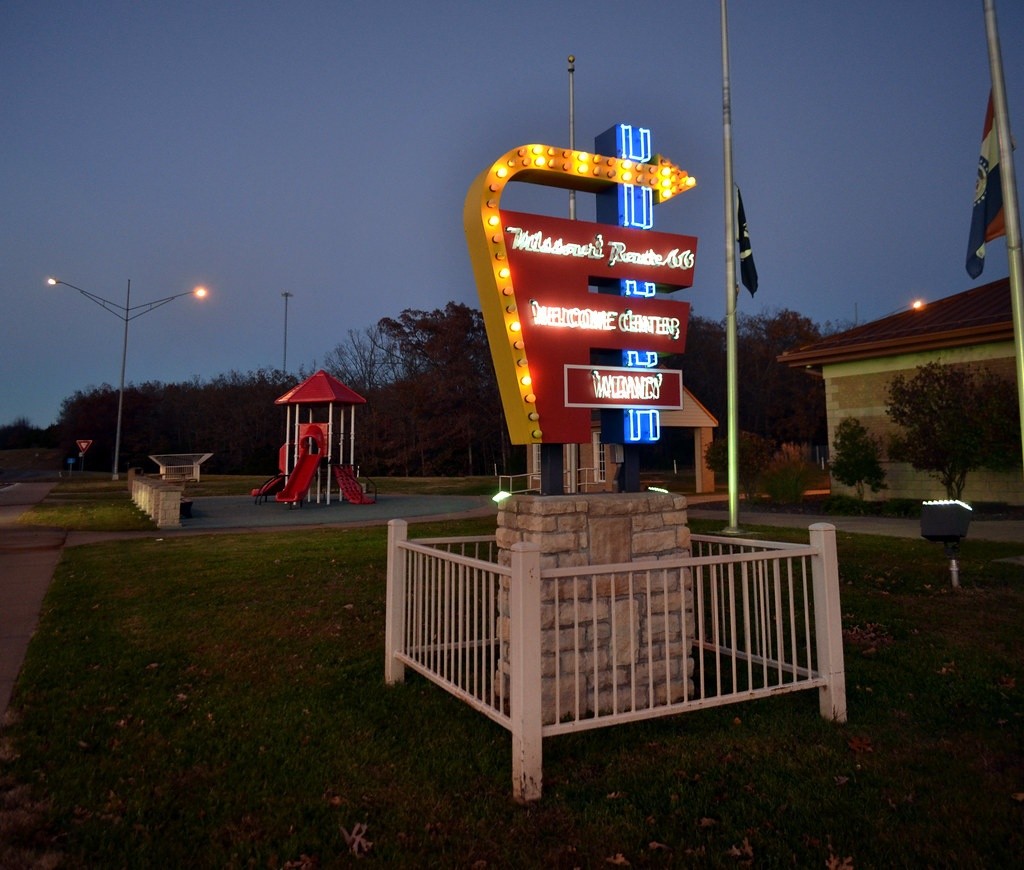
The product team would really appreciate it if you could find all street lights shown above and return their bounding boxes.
[282,291,294,382]
[46,278,208,480]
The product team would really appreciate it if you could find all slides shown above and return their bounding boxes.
[251,471,284,498]
[274,451,321,502]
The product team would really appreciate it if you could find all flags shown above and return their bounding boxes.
[733,191,759,301]
[965,83,1009,281]
